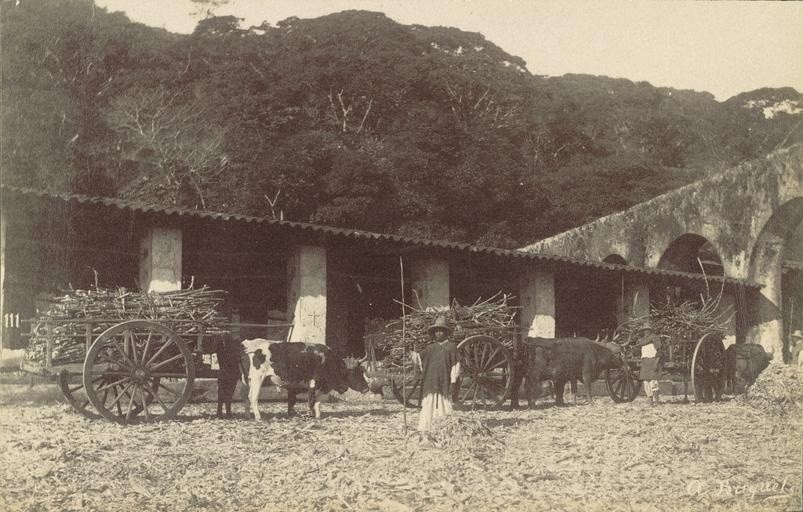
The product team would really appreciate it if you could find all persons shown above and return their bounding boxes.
[788,329,802,366]
[637,322,664,405]
[402,317,462,446]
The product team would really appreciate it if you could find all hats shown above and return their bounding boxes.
[641,322,653,331]
[427,315,452,338]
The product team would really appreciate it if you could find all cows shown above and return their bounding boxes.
[234,337,371,424]
[523,334,622,407]
[508,336,566,408]
[726,341,775,396]
[214,335,301,418]
